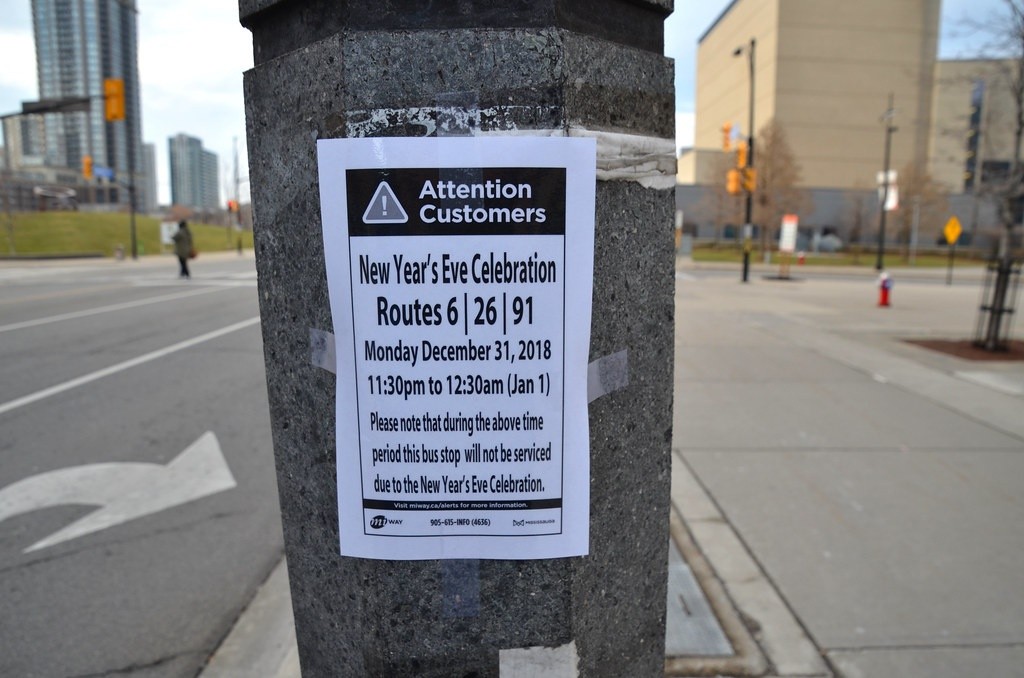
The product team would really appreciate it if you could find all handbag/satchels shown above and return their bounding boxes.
[189,249,198,258]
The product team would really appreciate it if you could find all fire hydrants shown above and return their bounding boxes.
[880,274,892,308]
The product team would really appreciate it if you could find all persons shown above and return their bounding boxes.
[172,220,195,280]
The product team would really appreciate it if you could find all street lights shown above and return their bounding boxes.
[730,37,757,283]
[875,94,898,274]
